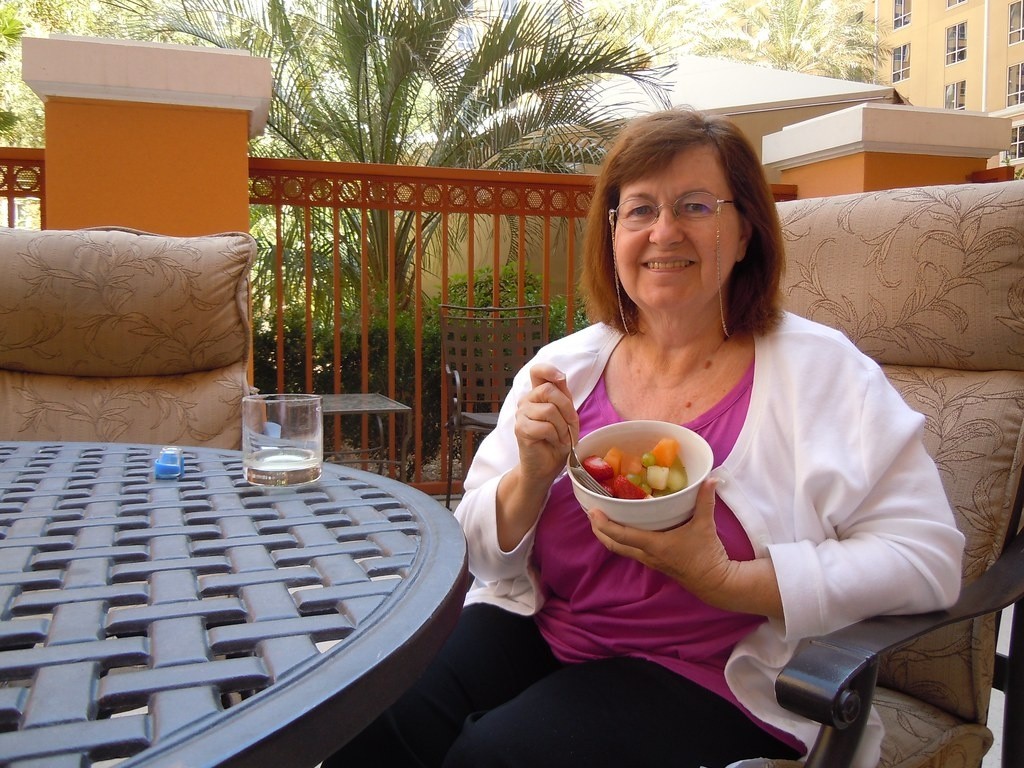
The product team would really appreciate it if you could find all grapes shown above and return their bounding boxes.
[626,451,655,494]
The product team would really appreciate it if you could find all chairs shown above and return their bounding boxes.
[0,226,257,451]
[439,304,546,511]
[714,178,1024,768]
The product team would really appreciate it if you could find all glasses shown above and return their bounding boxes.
[607,191,740,231]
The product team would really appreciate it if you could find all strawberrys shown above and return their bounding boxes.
[583,455,647,500]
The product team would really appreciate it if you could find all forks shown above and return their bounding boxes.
[567,426,614,498]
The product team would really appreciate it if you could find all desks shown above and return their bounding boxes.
[319,392,412,483]
[0,442,471,768]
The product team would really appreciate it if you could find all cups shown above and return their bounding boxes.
[242,394,323,487]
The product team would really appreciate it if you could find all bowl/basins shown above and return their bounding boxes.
[567,419,714,531]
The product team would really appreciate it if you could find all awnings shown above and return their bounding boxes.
[417,54,914,143]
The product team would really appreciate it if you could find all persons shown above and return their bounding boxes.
[387,102,965,768]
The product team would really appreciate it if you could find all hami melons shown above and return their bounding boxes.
[603,447,626,475]
[652,438,680,466]
